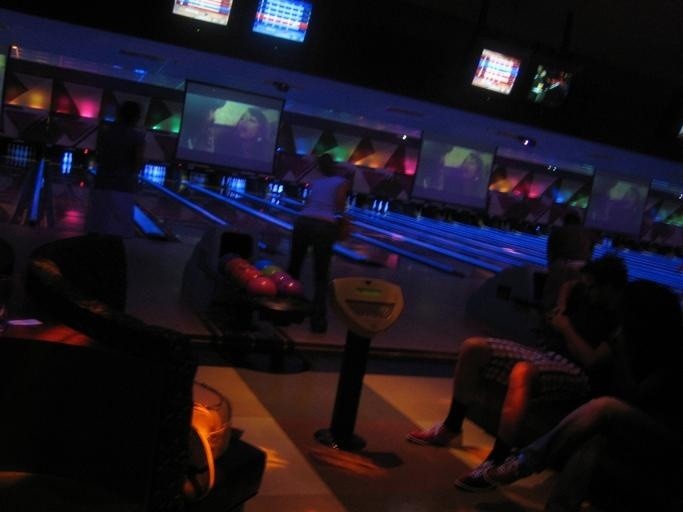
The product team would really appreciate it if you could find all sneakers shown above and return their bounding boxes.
[406,422,532,492]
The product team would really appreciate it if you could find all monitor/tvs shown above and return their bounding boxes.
[245,0,314,49]
[469,46,520,96]
[521,59,576,110]
[166,0,240,32]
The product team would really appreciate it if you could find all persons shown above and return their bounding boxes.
[83,100,148,240]
[410,141,495,201]
[291,152,351,335]
[585,172,641,238]
[177,93,274,158]
[406,210,682,512]
